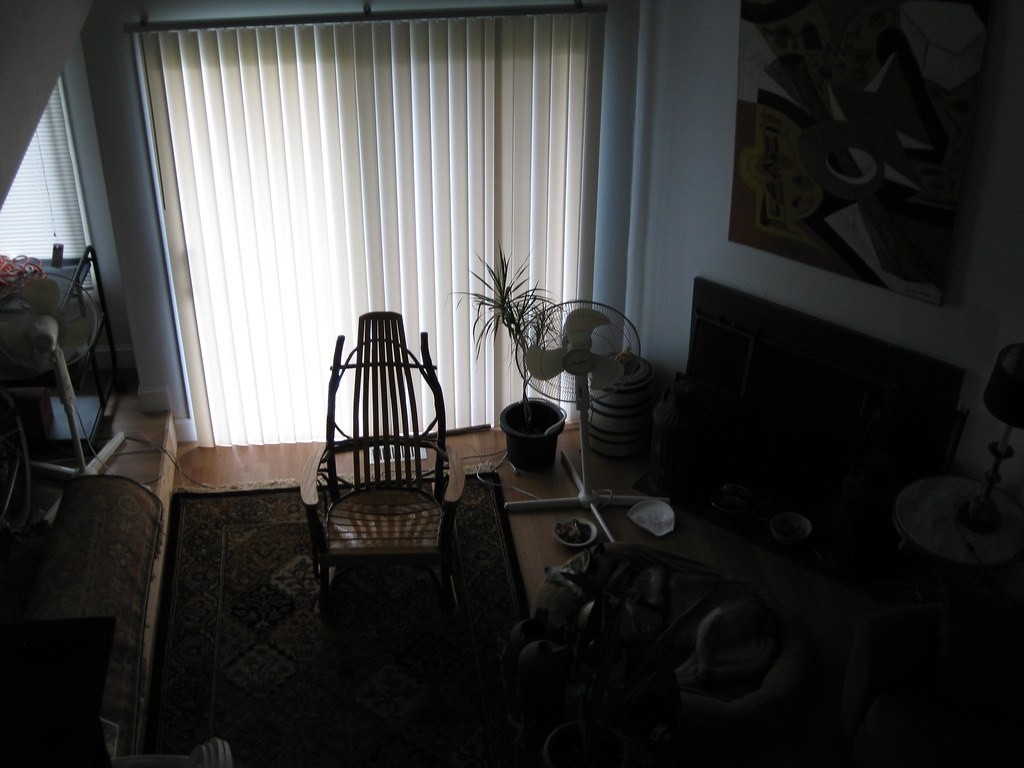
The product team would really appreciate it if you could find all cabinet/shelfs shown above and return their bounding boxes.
[1,244,121,454]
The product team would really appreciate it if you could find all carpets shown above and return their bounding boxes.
[0,474,164,760]
[145,471,566,768]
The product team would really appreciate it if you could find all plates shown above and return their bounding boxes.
[552,516,597,547]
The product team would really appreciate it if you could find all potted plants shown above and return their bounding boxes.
[540,591,633,768]
[456,238,565,473]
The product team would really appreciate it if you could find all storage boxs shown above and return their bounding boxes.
[1,388,54,440]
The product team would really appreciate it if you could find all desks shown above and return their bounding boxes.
[891,473,1024,606]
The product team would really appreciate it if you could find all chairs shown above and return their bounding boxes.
[299,311,465,619]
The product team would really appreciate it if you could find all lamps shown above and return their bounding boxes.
[957,342,1024,532]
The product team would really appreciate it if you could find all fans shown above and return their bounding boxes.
[504,300,669,546]
[1,272,152,526]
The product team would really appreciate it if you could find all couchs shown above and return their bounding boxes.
[838,598,1023,768]
